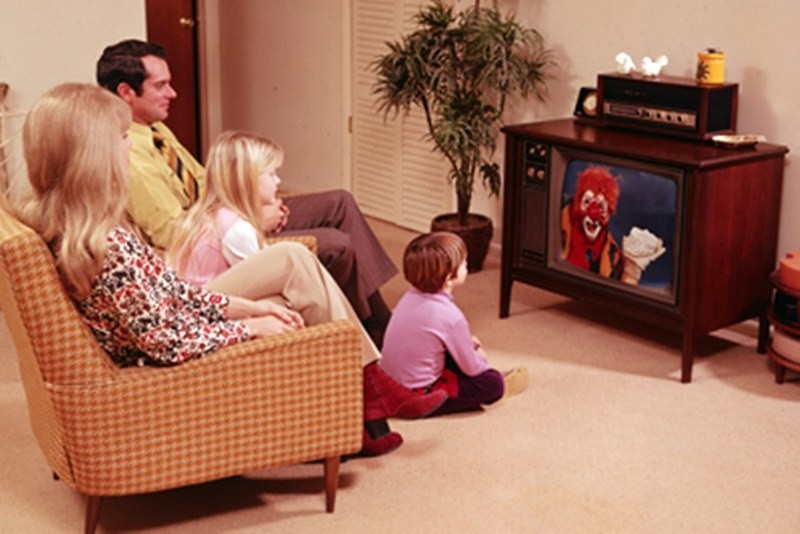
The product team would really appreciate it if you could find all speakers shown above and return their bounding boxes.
[595,73,739,141]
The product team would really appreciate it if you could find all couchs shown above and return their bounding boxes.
[0,209,364,534]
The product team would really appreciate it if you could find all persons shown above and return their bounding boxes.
[96,40,400,353]
[377,231,529,418]
[561,165,666,287]
[12,82,404,462]
[165,130,285,284]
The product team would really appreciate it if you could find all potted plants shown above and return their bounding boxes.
[364,0,569,273]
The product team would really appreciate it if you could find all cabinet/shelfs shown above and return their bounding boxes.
[498,116,789,383]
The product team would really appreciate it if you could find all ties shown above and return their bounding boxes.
[152,125,198,211]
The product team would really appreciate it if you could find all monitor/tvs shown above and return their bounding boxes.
[546,145,684,305]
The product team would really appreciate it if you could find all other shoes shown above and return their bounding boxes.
[503,366,529,397]
[361,424,403,456]
[361,363,447,422]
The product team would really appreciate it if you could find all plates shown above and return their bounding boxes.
[713,135,767,148]
